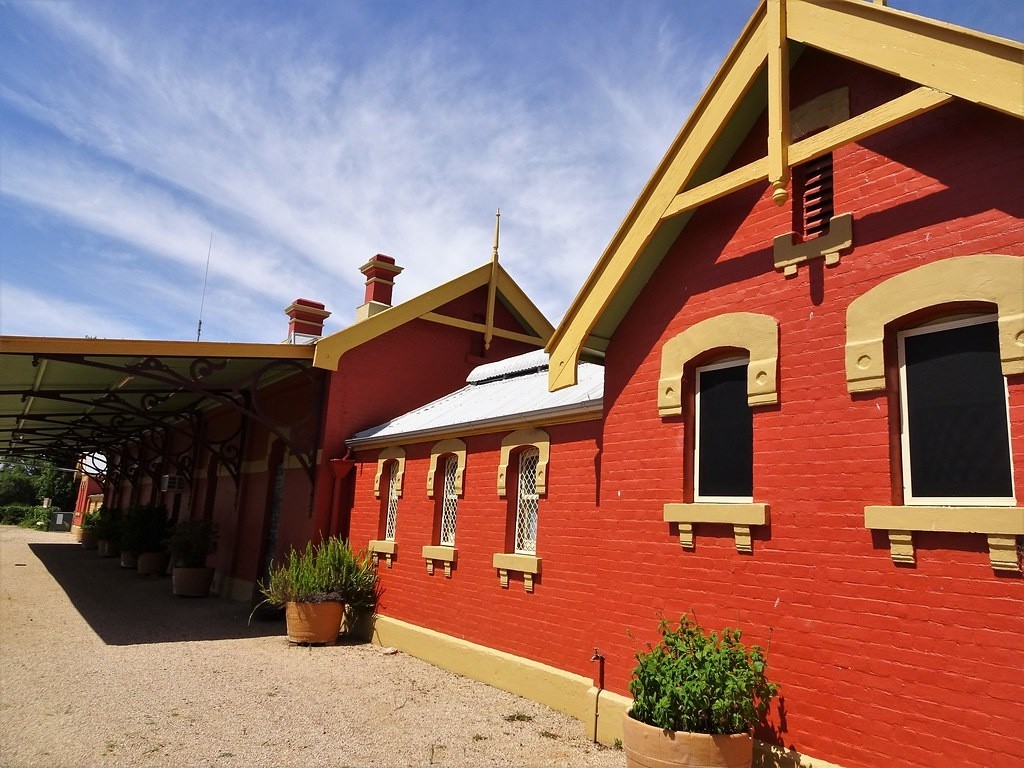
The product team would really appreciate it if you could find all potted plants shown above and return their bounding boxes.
[247,530,380,646]
[162,519,221,597]
[80,503,171,576]
[622,609,781,768]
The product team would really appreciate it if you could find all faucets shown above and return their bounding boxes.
[590,647,604,663]
